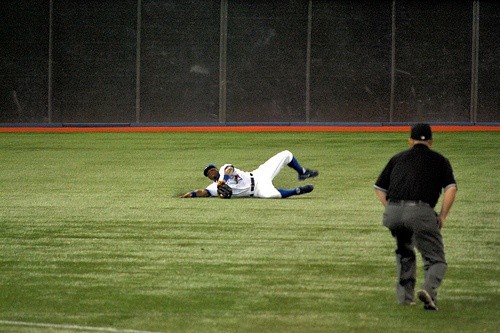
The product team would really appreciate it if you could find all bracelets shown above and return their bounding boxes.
[190,190,197,196]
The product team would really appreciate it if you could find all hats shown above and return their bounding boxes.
[204,163,216,176]
[411,123,432,141]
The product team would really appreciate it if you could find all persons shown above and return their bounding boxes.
[179,149,318,198]
[373,122,457,310]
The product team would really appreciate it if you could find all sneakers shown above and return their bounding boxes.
[396,300,417,306]
[416,289,441,311]
[296,184,314,195]
[299,170,319,181]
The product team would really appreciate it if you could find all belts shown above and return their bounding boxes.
[387,198,434,209]
[250,172,254,198]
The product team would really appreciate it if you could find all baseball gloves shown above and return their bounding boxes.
[217,182,233,199]
